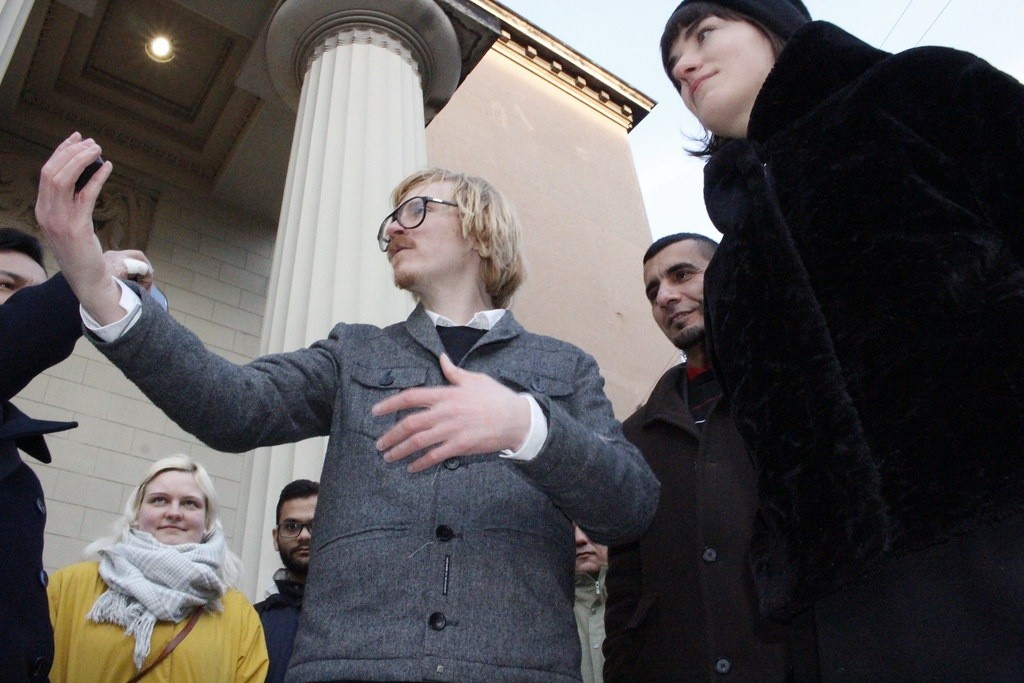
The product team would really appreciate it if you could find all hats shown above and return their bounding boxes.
[662,0,812,96]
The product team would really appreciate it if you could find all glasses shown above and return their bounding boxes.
[277,517,312,538]
[377,196,458,252]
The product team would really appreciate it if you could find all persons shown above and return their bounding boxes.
[34,126,664,683]
[602,233,790,681]
[659,0,1024,683]
[0,225,154,683]
[43,453,270,683]
[250,480,320,683]
[572,517,610,683]
